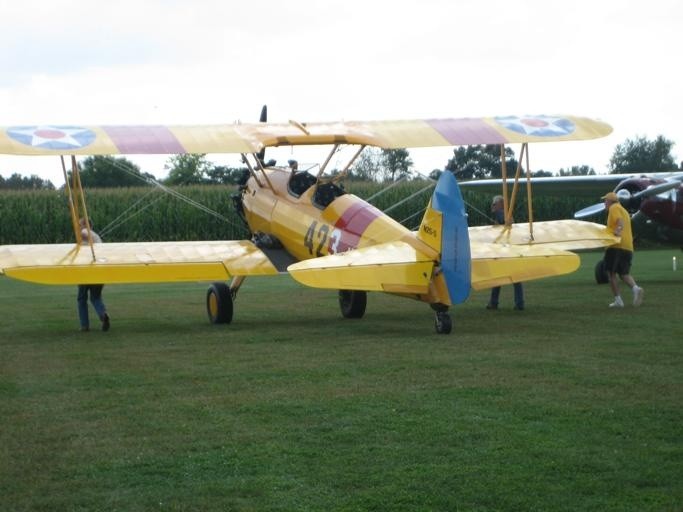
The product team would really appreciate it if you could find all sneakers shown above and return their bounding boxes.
[633,288,642,307]
[609,302,624,308]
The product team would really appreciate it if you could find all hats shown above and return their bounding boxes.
[600,192,617,201]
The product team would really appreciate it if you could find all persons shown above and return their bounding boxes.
[485,194,526,311]
[76,216,111,332]
[600,191,644,308]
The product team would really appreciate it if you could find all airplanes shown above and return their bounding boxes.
[456,170,682,284]
[0,106,624,335]
